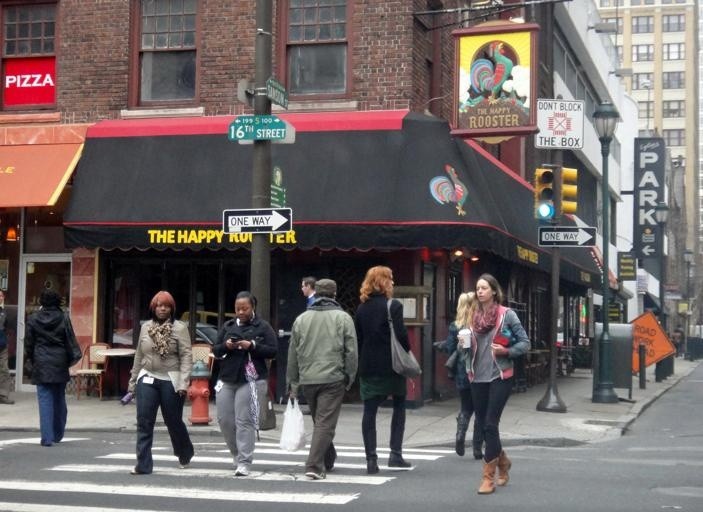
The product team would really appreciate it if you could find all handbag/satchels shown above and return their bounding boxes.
[389,326,423,377]
[69,343,82,367]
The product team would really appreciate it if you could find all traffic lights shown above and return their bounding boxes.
[534,168,553,219]
[562,167,577,214]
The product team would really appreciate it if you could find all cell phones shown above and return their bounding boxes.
[230,337,238,343]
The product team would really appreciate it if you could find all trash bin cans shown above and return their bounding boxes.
[594,321,637,405]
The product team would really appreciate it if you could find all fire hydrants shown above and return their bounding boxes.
[186,359,215,426]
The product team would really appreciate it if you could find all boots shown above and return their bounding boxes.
[472,430,484,460]
[477,458,499,494]
[454,415,468,457]
[366,455,380,473]
[497,450,512,486]
[388,452,413,467]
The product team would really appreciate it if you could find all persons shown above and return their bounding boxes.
[671,329,681,357]
[456,272,531,493]
[0,289,14,404]
[445,292,484,459]
[23,287,82,446]
[212,291,279,476]
[125,289,194,475]
[284,278,359,479]
[674,324,685,357]
[353,265,421,474]
[300,277,316,310]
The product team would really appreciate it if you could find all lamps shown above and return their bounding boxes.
[6,225,16,242]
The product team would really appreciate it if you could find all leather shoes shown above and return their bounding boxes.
[130,468,138,474]
[179,463,189,469]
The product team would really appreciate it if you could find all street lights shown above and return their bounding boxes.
[591,100,622,405]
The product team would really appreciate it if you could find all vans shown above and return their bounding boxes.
[179,309,235,329]
[4,304,70,368]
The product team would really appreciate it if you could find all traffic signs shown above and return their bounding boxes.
[538,226,597,248]
[262,76,288,110]
[226,111,287,143]
[222,207,292,234]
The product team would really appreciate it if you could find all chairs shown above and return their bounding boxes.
[72,342,111,401]
[190,343,215,386]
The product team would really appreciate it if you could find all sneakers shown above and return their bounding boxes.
[304,465,328,479]
[233,455,250,476]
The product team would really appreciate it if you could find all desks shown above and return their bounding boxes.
[97,348,136,399]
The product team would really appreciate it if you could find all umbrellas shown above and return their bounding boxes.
[121,391,134,406]
[245,339,261,442]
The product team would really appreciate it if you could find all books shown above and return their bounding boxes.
[168,370,188,393]
